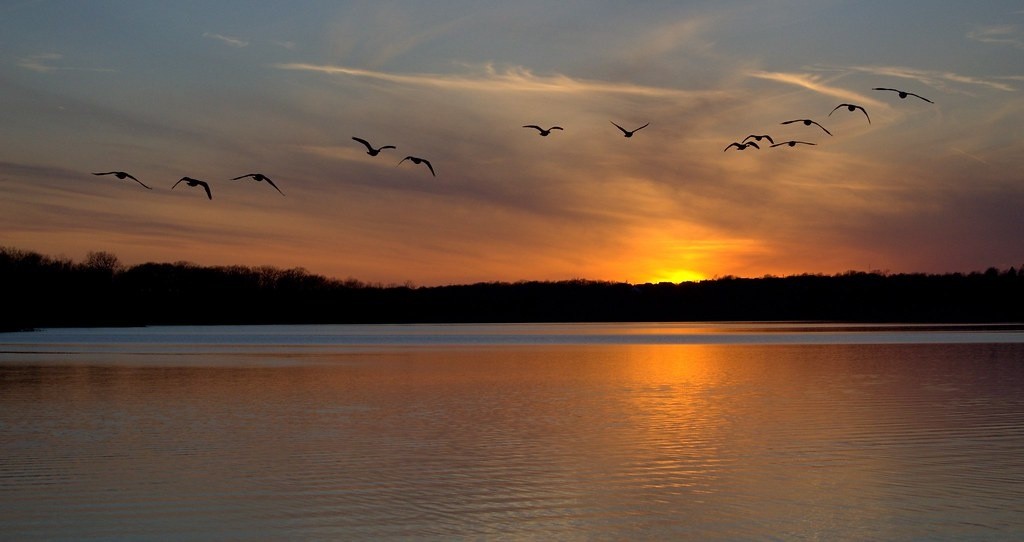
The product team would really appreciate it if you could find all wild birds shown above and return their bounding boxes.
[609,118,651,139]
[521,123,565,137]
[350,136,437,178]
[723,86,935,154]
[91,170,287,202]
